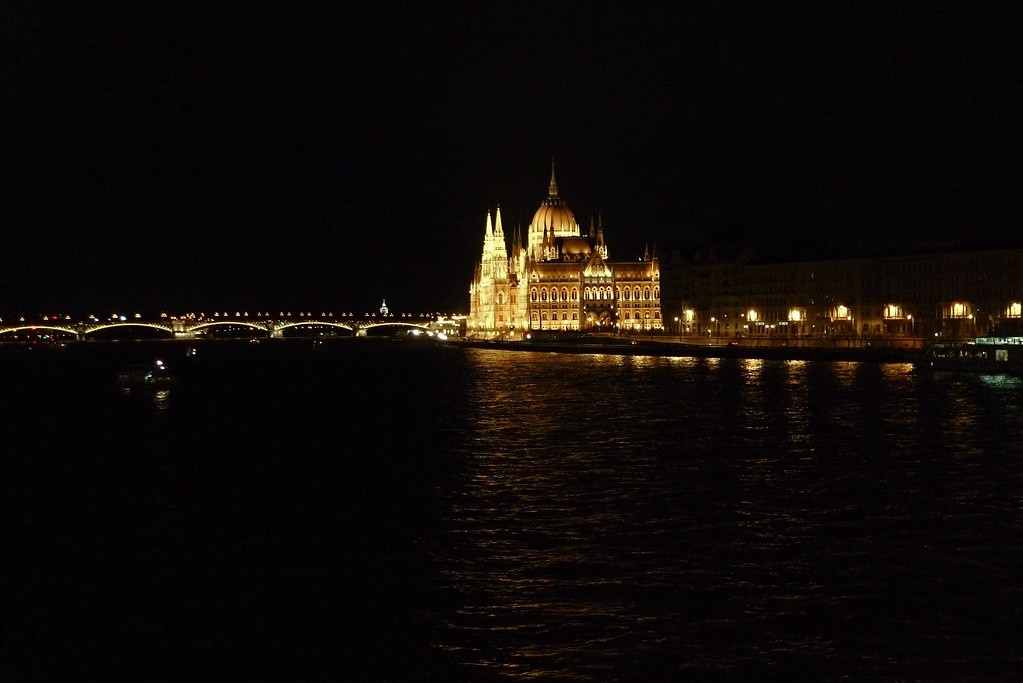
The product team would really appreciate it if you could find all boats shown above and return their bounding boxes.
[918,335,1023,374]
